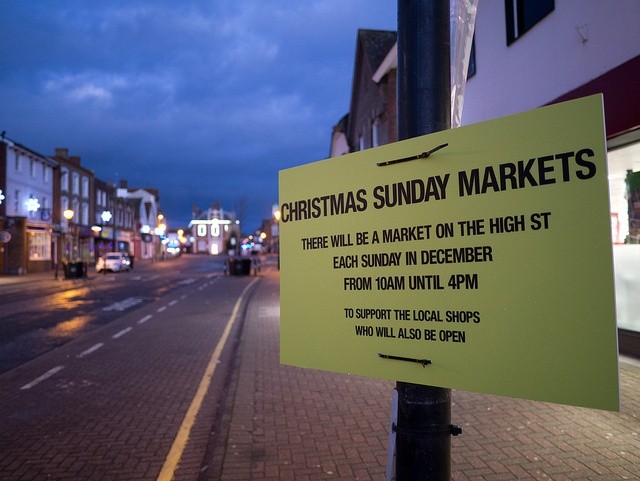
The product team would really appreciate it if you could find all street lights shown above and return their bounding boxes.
[98,211,113,274]
[275,210,281,270]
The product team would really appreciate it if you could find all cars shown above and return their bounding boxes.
[96,252,131,271]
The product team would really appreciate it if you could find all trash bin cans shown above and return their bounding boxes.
[64,261,86,279]
[229,257,251,275]
